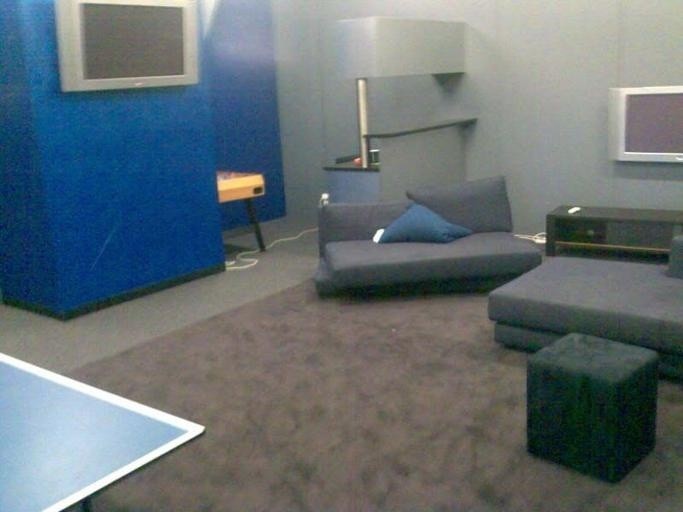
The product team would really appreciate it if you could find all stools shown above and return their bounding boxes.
[525,332,658,483]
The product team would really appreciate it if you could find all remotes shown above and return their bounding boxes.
[569,206,581,214]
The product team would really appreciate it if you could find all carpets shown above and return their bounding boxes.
[53,271,682,506]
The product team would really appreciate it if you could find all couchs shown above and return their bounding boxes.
[314,175,541,299]
[485,235,681,381]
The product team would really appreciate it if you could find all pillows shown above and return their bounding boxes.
[377,203,473,244]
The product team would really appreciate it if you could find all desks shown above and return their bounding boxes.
[545,205,682,257]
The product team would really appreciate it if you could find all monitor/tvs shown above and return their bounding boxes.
[608,85,683,162]
[54,1,200,92]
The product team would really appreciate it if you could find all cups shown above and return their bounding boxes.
[368,149,379,163]
